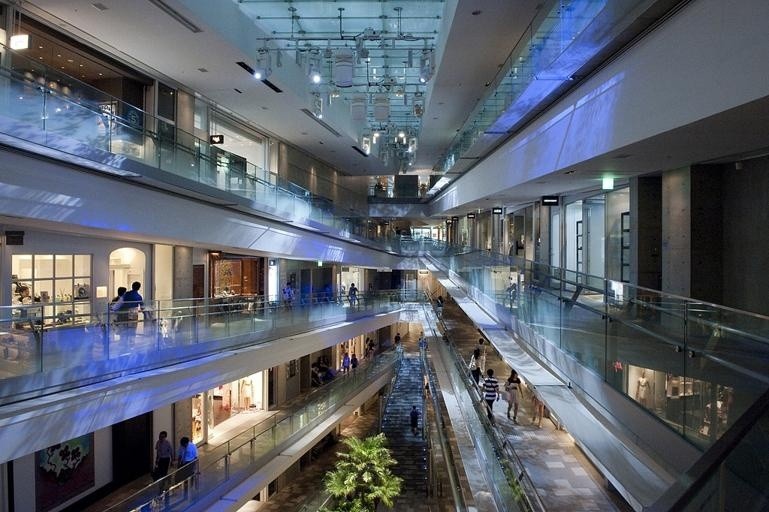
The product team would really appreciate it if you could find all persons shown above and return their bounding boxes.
[240,375,254,411]
[410,405,421,436]
[109,281,153,348]
[351,354,358,370]
[367,339,378,360]
[111,286,127,306]
[349,283,360,305]
[468,347,482,391]
[365,337,370,358]
[174,437,199,497]
[636,370,651,407]
[282,282,293,313]
[504,369,524,423]
[436,295,445,319]
[530,392,545,429]
[12,286,36,332]
[342,353,350,377]
[151,430,174,501]
[475,337,486,376]
[482,369,500,421]
[508,240,524,256]
[394,332,400,348]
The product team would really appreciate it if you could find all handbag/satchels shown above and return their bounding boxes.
[471,362,477,370]
[502,391,510,400]
[152,467,161,481]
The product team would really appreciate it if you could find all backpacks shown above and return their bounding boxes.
[110,296,123,310]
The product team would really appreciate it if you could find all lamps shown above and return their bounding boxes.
[252,6,436,176]
[96,286,108,298]
[602,177,615,190]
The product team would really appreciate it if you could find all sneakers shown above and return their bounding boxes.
[507,412,518,424]
[531,421,542,429]
